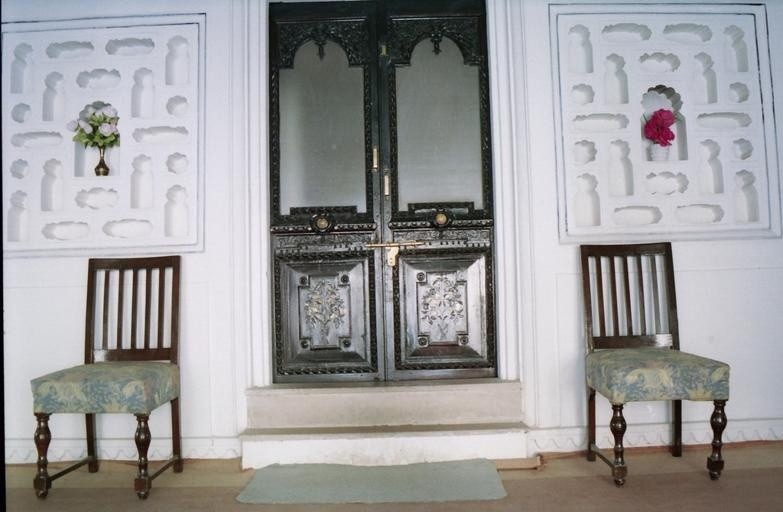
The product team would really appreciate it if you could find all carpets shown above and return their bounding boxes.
[236,458,508,504]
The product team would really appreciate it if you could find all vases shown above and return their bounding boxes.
[94,149,109,176]
[651,146,669,161]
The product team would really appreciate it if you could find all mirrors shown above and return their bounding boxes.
[383,33,487,218]
[269,35,367,214]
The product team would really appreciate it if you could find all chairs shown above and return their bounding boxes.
[579,241,729,487]
[32,255,181,502]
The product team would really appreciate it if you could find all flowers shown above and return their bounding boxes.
[642,108,677,146]
[67,103,121,149]
